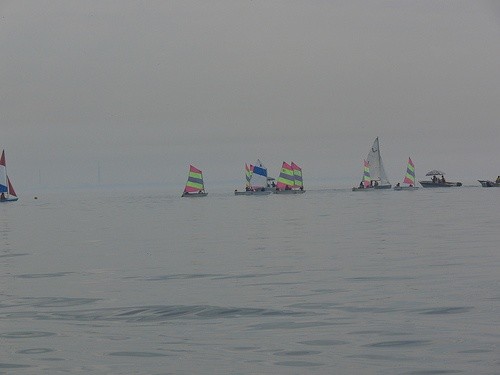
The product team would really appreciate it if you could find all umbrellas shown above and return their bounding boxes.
[425,168,445,176]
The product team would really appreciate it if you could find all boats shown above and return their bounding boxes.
[476,179,500,187]
[418,170,463,187]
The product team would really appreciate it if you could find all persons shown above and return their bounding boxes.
[246,180,294,191]
[361,180,379,188]
[431,175,446,183]
[396,183,400,188]
[1,193,6,200]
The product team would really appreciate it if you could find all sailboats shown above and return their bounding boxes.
[392,156,420,190]
[0,150,18,201]
[181,164,208,197]
[234,158,305,194]
[352,136,391,190]
